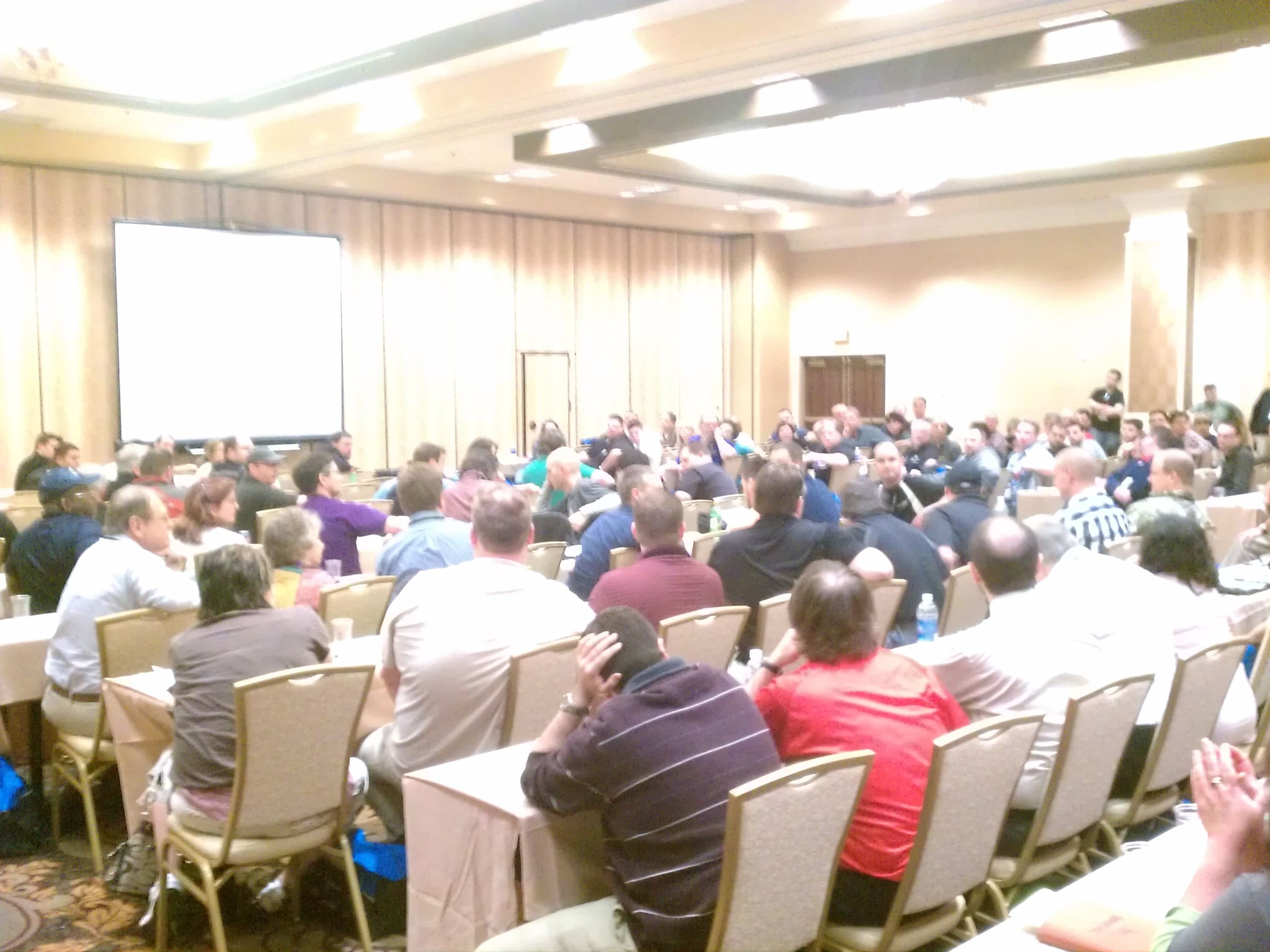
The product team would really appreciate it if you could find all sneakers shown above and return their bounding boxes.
[139,873,182,942]
[245,867,289,914]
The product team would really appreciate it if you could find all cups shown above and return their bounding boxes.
[332,615,353,657]
[325,559,341,583]
[8,595,32,628]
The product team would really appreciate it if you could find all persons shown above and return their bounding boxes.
[1250,387,1270,434]
[1186,384,1243,430]
[1089,369,1125,456]
[0,396,1270,950]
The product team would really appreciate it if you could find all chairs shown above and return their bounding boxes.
[497,629,597,748]
[760,591,808,673]
[827,707,1047,952]
[957,668,1154,915]
[260,432,882,589]
[318,575,397,641]
[658,605,754,675]
[46,603,208,874]
[156,661,379,952]
[1086,635,1255,843]
[936,563,995,636]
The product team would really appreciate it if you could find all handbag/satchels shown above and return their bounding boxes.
[103,821,157,896]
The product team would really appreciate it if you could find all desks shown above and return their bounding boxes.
[1018,485,1066,521]
[104,619,400,843]
[399,639,928,952]
[0,610,61,794]
[1195,488,1270,566]
